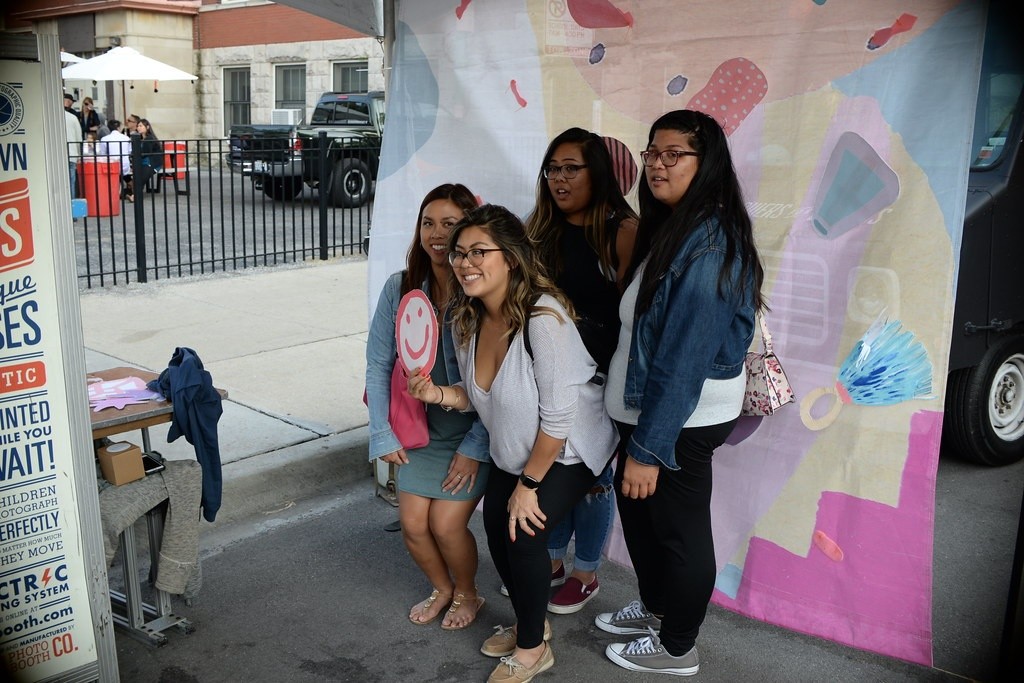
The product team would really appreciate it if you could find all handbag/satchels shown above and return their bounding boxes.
[389,356,429,450]
[742,353,796,417]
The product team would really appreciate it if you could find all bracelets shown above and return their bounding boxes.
[436,384,461,412]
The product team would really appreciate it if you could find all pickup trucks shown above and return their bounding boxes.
[225,91,385,209]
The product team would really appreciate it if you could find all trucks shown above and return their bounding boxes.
[939,0,1024,468]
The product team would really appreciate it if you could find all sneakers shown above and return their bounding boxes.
[606,625,700,676]
[595,600,662,633]
[486,640,555,683]
[481,618,552,657]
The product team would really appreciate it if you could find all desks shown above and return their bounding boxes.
[80,367,228,649]
[149,150,191,196]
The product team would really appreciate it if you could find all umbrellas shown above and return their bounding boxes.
[61,47,198,137]
[59,51,88,62]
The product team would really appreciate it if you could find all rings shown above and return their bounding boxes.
[457,475,461,480]
[509,517,516,520]
[518,518,526,521]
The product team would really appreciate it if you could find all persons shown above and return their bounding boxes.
[63,93,164,203]
[594,111,771,676]
[500,128,641,612]
[365,183,491,629]
[406,203,621,683]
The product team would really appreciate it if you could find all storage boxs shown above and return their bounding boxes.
[97,441,145,485]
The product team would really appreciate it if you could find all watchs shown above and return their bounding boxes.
[520,471,541,489]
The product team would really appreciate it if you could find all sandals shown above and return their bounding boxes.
[409,588,454,624]
[442,587,486,629]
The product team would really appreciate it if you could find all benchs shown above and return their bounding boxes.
[153,168,195,194]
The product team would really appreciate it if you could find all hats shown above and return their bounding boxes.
[64,93,77,102]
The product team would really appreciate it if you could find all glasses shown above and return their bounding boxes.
[449,248,505,266]
[640,149,704,166]
[544,164,588,179]
[127,119,136,123]
[84,103,88,106]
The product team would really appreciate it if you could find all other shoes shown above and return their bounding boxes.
[547,572,599,615]
[500,562,566,596]
[126,194,134,203]
